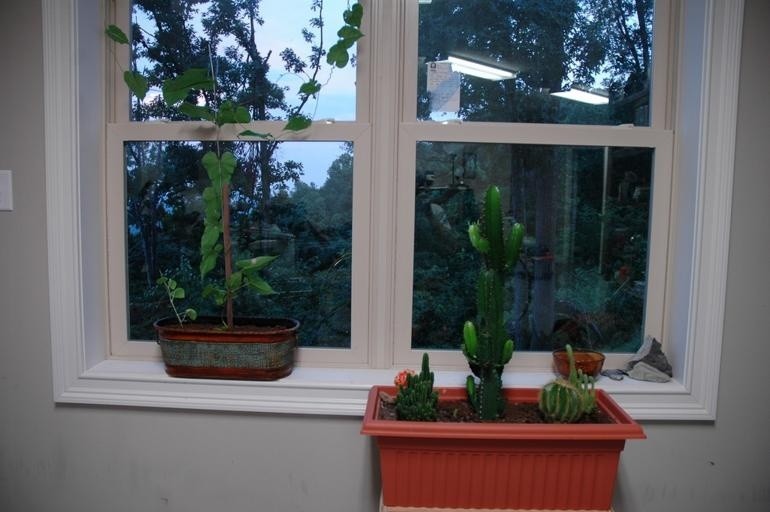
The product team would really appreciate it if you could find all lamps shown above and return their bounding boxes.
[548,83,611,106]
[439,49,521,82]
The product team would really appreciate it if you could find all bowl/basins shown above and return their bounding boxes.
[552,347,606,382]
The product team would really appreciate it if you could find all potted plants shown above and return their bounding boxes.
[104,3,366,382]
[359,182,646,510]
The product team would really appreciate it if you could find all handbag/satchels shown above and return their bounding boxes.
[601,336,673,383]
